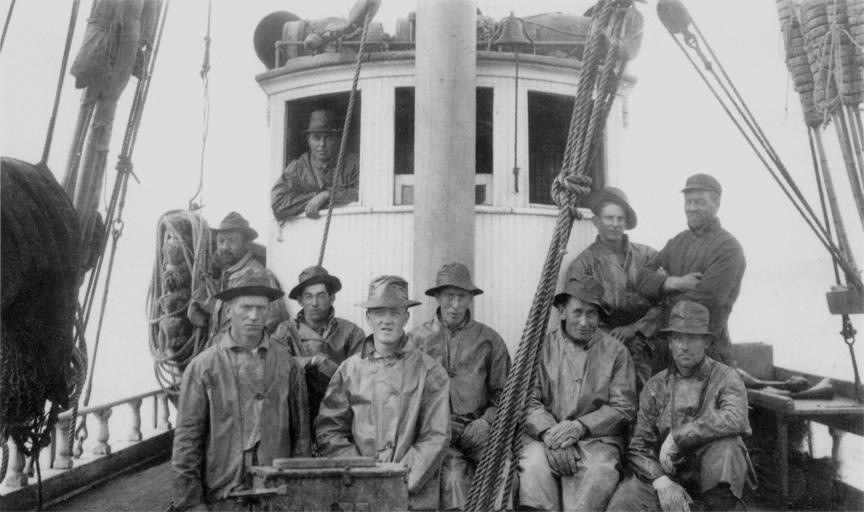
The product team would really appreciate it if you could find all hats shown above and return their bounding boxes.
[356,275,422,309]
[214,268,285,301]
[300,107,345,136]
[588,187,637,230]
[210,212,259,240]
[551,276,611,317]
[681,173,722,194]
[661,301,717,337]
[290,266,343,299]
[425,262,484,296]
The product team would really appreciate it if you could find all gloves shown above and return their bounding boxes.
[546,445,579,477]
[545,419,586,449]
[654,475,694,511]
[661,433,680,474]
[664,273,703,296]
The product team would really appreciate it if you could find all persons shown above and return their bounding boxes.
[313,275,450,510]
[606,299,755,512]
[409,265,513,511]
[639,171,746,378]
[567,185,662,382]
[271,265,363,417]
[185,212,272,342]
[170,268,311,512]
[517,276,635,512]
[269,107,360,221]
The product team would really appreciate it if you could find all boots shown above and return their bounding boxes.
[764,377,844,401]
[742,369,812,392]
